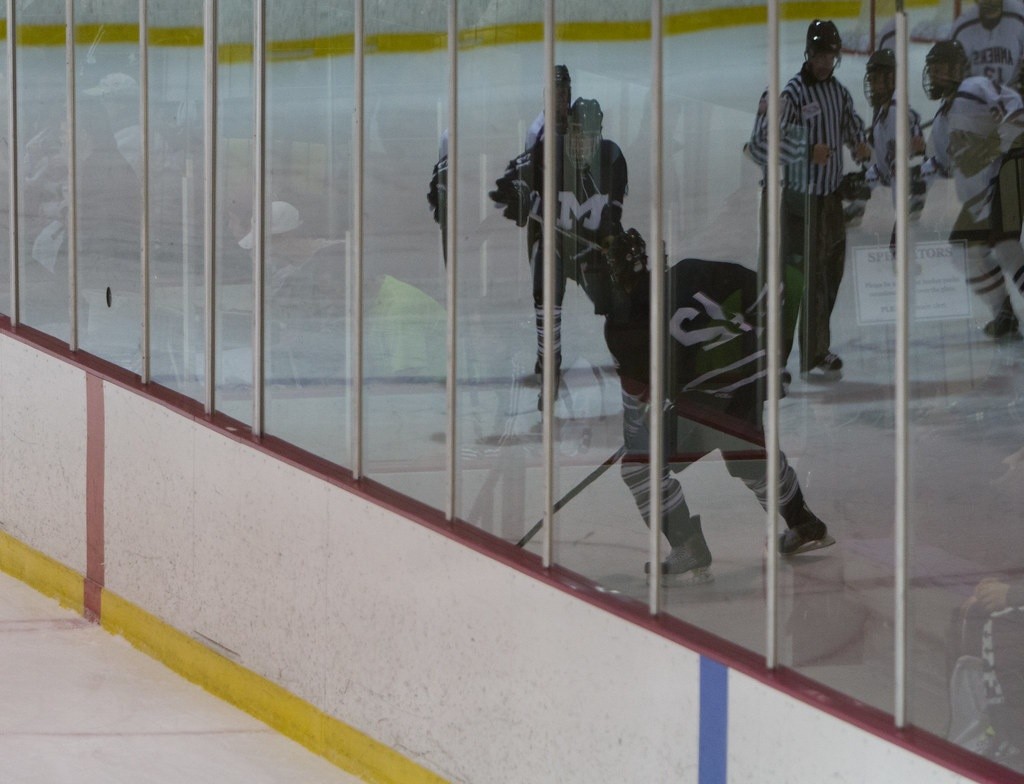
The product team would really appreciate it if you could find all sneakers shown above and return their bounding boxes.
[779,369,792,387]
[536,371,560,411]
[817,352,844,371]
[775,502,837,557]
[645,514,717,588]
[983,294,1019,337]
[844,203,865,227]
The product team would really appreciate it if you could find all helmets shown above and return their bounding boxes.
[437,128,450,159]
[804,18,842,58]
[926,39,967,66]
[553,63,572,103]
[866,48,895,73]
[563,95,604,170]
[569,220,647,315]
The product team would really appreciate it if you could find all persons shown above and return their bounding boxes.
[604,228,835,586]
[491,97,627,410]
[860,48,928,257]
[743,18,870,395]
[949,579,1024,771]
[949,0,1024,86]
[921,40,1024,338]
[1,0,1023,784]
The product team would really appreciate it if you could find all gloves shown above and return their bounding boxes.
[488,178,531,227]
